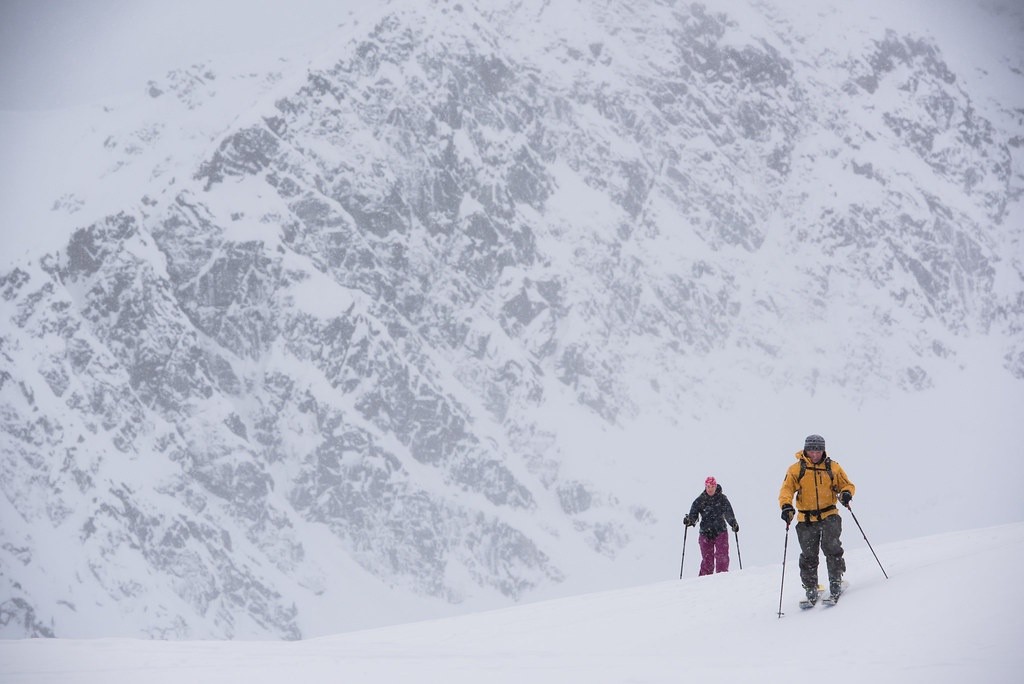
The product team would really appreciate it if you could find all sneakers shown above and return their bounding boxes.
[830,581,841,597]
[805,587,818,602]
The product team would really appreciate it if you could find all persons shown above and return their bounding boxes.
[682,476,740,577]
[778,433,855,604]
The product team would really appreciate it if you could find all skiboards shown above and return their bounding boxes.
[821,580,851,605]
[798,583,825,609]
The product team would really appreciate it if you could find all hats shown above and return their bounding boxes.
[704,477,717,486]
[805,435,826,450]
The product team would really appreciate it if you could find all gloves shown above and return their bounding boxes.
[731,523,740,531]
[781,505,795,521]
[683,515,692,525]
[841,491,852,507]
